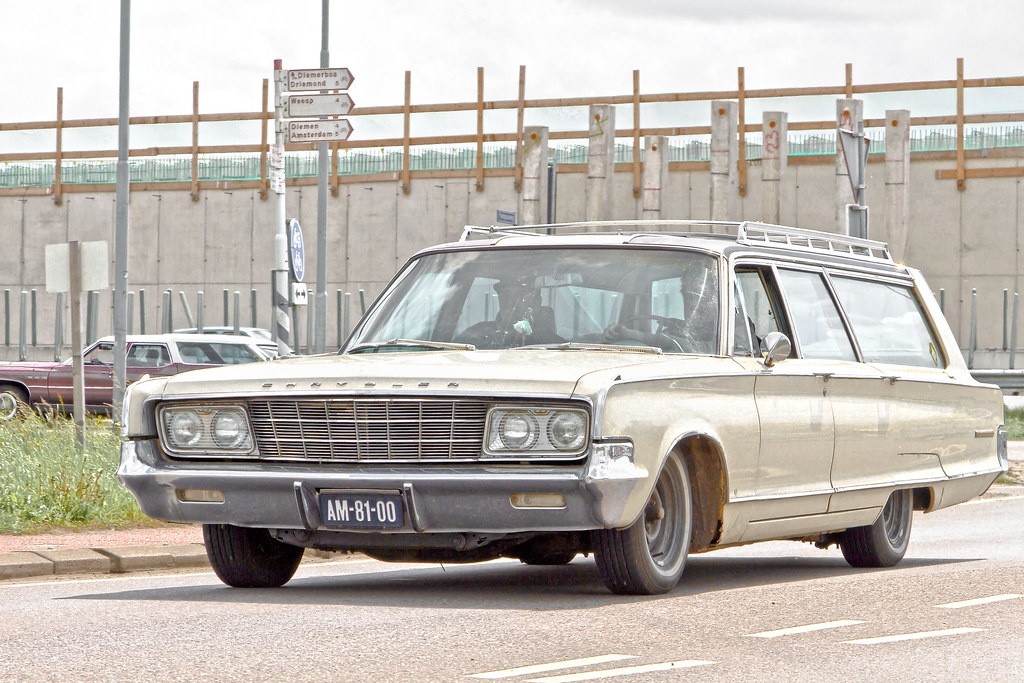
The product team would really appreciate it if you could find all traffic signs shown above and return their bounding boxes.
[288,68,356,143]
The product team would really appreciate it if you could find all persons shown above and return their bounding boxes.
[597,253,766,359]
[449,264,574,354]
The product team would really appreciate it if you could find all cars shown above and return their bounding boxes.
[174,326,297,360]
[114,220,1009,595]
[0,335,278,422]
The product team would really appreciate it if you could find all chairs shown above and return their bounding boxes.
[495,306,570,347]
